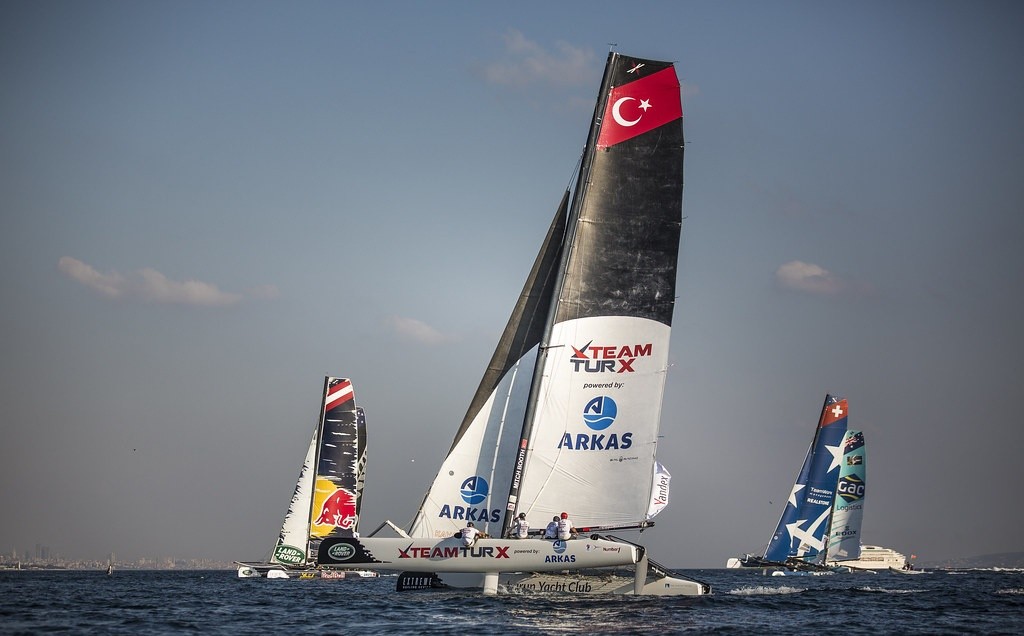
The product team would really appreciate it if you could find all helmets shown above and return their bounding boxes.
[519,513,526,518]
[467,522,474,526]
[553,516,560,522]
[561,513,568,519]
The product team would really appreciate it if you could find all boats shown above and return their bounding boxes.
[837,540,907,570]
[107,563,112,576]
[888,554,934,574]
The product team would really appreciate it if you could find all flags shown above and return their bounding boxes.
[911,555,917,559]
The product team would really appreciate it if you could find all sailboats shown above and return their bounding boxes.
[726,393,885,580]
[312,51,714,602]
[235,375,382,579]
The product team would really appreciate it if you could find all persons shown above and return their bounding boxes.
[513,513,530,539]
[462,522,486,548]
[906,562,910,570]
[544,516,561,540]
[558,513,578,541]
